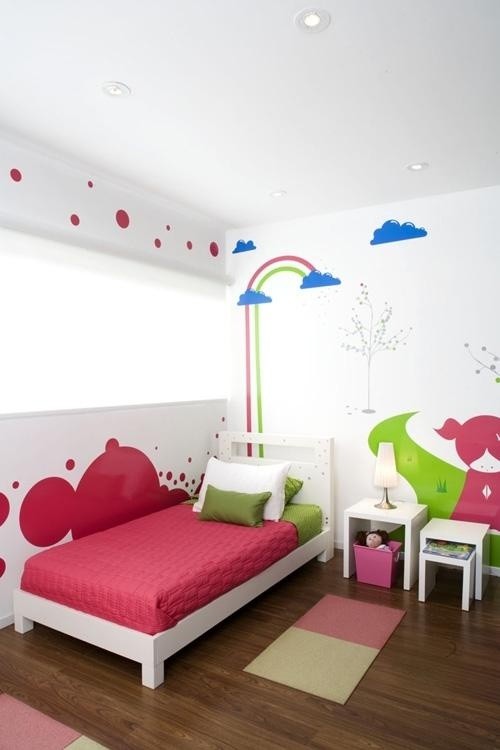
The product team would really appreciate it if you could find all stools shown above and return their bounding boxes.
[417,541,477,611]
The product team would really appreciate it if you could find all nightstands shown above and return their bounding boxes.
[343,500,428,591]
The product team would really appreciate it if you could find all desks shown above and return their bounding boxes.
[420,518,491,603]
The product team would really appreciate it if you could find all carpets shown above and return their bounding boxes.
[242,592,405,706]
[0,693,113,749]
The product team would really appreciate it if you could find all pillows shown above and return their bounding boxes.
[192,457,304,526]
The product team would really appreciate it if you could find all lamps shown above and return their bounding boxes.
[374,442,400,510]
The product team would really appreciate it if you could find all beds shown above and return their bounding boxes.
[14,430,334,690]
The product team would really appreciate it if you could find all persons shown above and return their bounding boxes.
[355,528,393,552]
